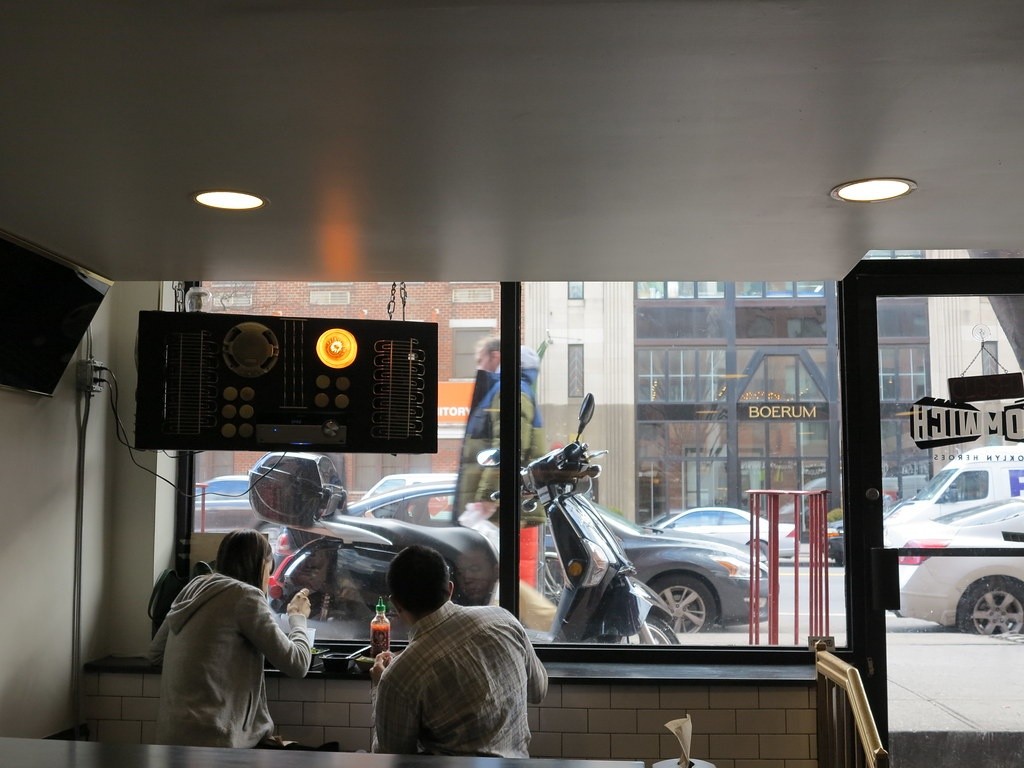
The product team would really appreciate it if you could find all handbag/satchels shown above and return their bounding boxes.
[146,560,214,639]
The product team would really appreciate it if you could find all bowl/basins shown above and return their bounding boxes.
[308,647,329,670]
[356,655,386,673]
[317,652,361,673]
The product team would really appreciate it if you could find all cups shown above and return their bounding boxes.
[281,614,291,633]
[305,628,317,650]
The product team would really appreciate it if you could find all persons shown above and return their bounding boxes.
[145,528,312,750]
[367,546,548,759]
[454,542,499,600]
[450,336,547,534]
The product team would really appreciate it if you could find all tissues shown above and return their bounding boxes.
[652,713,717,768]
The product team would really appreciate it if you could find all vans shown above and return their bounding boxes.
[882,443,1024,542]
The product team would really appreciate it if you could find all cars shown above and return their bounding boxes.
[649,507,798,563]
[335,474,774,636]
[826,517,845,567]
[894,495,1024,635]
[192,475,286,555]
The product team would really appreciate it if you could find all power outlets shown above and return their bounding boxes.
[77,359,104,392]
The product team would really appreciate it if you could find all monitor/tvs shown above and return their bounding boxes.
[0,231,115,397]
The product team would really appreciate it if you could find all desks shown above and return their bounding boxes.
[0,736,647,768]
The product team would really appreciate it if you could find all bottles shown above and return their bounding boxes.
[185,287,212,313]
[370,596,392,658]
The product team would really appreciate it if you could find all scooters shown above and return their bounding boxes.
[246,393,682,646]
